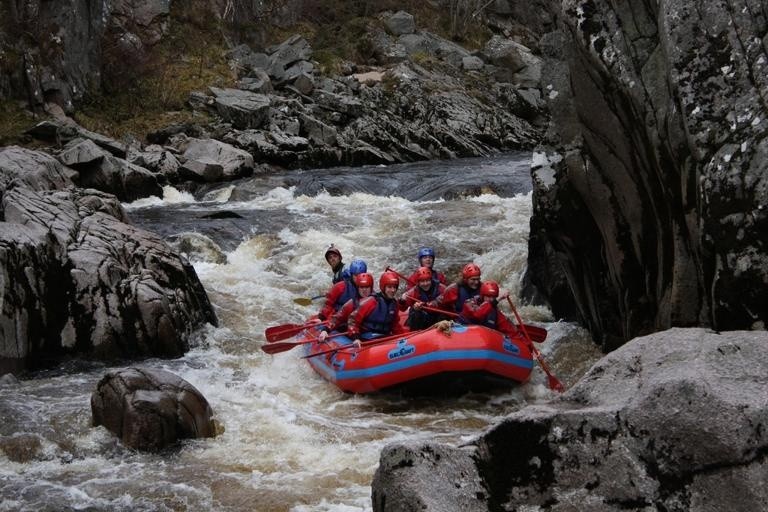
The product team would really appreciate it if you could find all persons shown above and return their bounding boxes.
[397,266,459,332]
[405,248,451,291]
[318,273,377,343]
[307,261,369,325]
[347,269,403,348]
[320,246,353,298]
[458,279,541,361]
[413,262,483,322]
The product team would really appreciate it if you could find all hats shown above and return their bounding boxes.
[325,248,343,258]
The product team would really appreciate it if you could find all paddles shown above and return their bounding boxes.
[260,332,350,354]
[294,294,328,306]
[303,329,420,359]
[506,294,564,393]
[420,305,548,343]
[266,320,330,342]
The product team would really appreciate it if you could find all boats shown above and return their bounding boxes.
[300,309,534,391]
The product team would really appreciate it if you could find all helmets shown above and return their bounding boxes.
[379,272,400,291]
[462,264,481,283]
[342,268,354,280]
[350,260,368,275]
[416,267,432,282]
[354,273,374,286]
[418,248,436,259]
[480,281,499,301]
